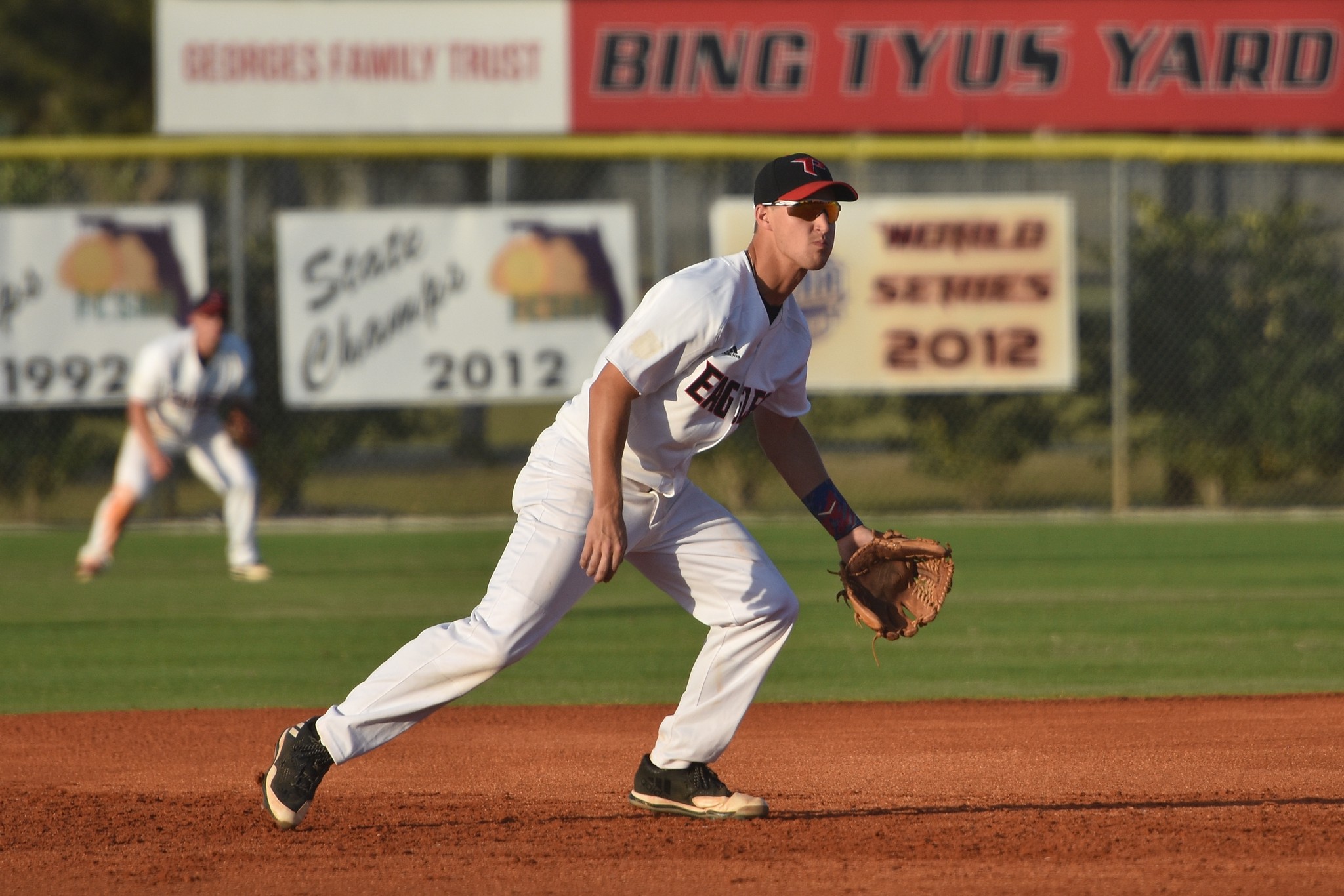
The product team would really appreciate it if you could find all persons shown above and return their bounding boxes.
[264,154,953,834]
[76,288,277,587]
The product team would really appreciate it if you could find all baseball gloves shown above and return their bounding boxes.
[230,408,258,449]
[824,526,955,666]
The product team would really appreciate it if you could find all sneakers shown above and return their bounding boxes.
[262,715,338,829]
[627,749,769,819]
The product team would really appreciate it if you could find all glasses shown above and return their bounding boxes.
[760,198,842,225]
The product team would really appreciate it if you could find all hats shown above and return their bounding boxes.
[177,288,227,325]
[752,153,857,207]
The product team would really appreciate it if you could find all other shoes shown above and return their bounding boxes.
[228,564,271,582]
